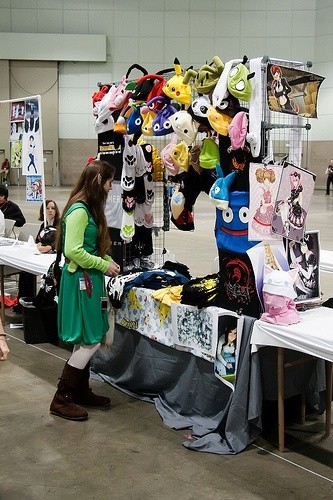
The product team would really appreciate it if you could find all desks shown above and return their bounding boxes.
[0,237,333,453]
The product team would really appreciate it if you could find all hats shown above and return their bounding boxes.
[140,105,159,137]
[159,139,179,175]
[109,75,135,111]
[228,112,250,152]
[126,63,184,101]
[147,97,180,136]
[199,139,220,169]
[216,90,249,117]
[171,193,195,232]
[162,57,193,104]
[127,107,144,134]
[170,141,191,172]
[207,107,233,136]
[188,146,203,175]
[183,56,224,93]
[262,265,299,299]
[187,92,213,128]
[209,164,239,211]
[136,75,173,112]
[228,58,256,102]
[92,105,115,134]
[168,109,201,144]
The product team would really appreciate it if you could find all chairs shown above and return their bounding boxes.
[4,219,42,242]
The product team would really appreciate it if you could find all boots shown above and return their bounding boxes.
[49,360,88,420]
[73,362,111,407]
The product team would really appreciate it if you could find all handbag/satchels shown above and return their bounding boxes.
[32,259,63,312]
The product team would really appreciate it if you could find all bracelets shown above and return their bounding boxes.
[0,334,6,336]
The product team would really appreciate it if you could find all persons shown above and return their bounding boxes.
[1,158,10,186]
[0,317,9,361]
[0,184,26,226]
[325,159,333,195]
[50,162,120,421]
[14,200,60,313]
